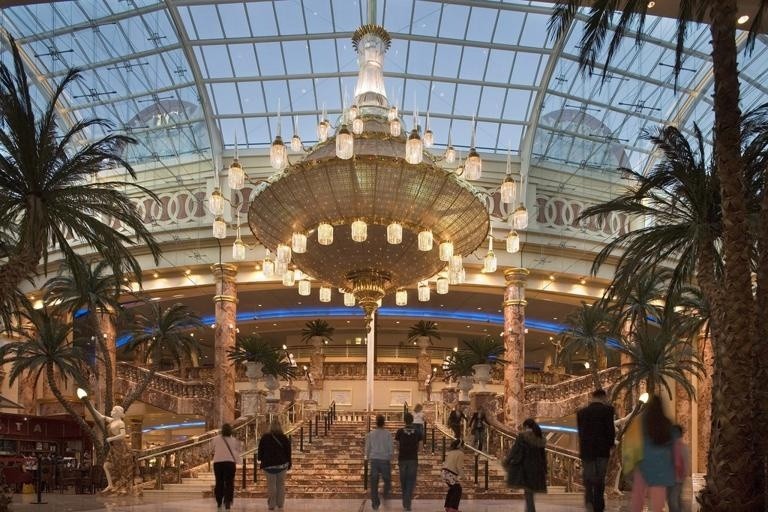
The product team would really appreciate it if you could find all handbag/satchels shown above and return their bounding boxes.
[639,446,674,486]
[508,456,523,485]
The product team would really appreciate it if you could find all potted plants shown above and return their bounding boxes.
[406,319,441,354]
[299,318,339,350]
[224,333,298,397]
[440,333,509,398]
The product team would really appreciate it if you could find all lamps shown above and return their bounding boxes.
[210,1,531,323]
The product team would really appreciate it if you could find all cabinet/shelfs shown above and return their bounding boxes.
[0,412,94,494]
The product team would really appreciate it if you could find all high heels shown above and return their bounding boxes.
[445,507,462,512]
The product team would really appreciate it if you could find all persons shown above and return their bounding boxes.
[576,389,617,511]
[442,438,465,512]
[447,404,468,446]
[500,418,548,512]
[670,425,689,512]
[209,423,238,512]
[256,419,290,511]
[92,405,127,493]
[394,413,424,512]
[468,406,490,451]
[364,415,393,510]
[622,395,676,512]
[411,404,425,441]
